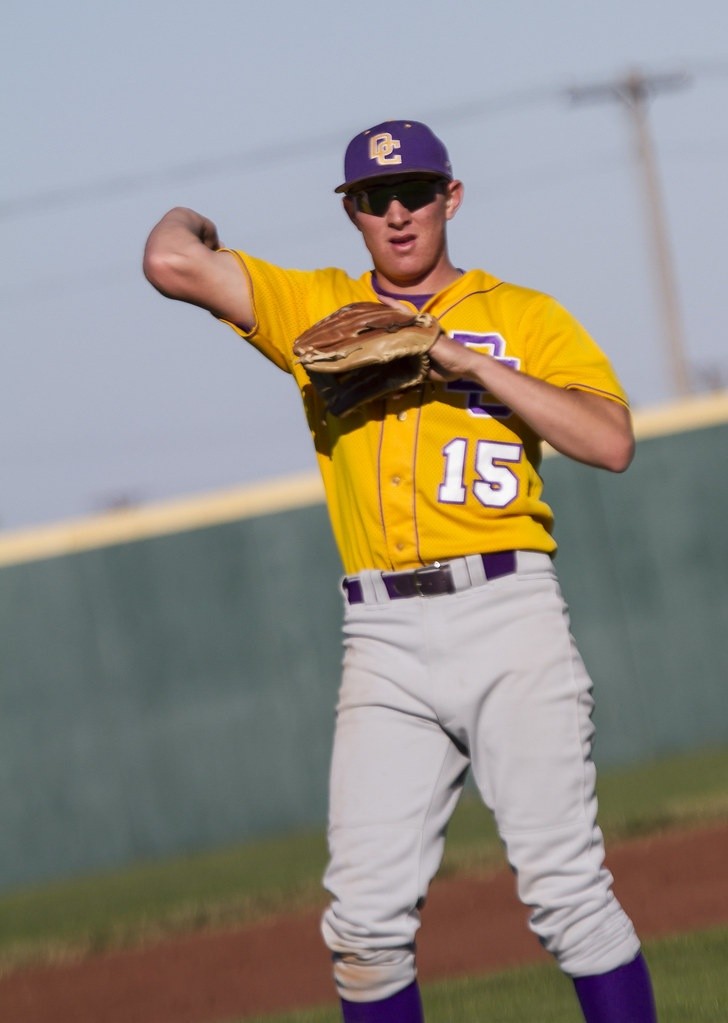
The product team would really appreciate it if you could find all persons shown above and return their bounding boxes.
[143,119,664,1023]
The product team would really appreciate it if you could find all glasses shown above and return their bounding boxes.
[350,178,452,217]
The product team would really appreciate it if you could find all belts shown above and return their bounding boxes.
[347,550,518,604]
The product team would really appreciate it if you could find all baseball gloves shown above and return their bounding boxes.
[292,301,447,419]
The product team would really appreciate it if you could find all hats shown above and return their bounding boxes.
[334,119,453,192]
